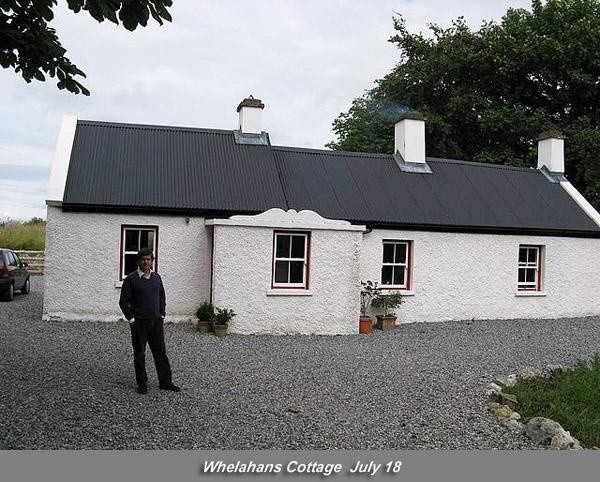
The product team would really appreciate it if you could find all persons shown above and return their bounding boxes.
[119,247,183,395]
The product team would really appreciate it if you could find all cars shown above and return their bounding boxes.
[0,249,30,302]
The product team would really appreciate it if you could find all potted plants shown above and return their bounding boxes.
[359,280,383,334]
[213,307,237,336]
[371,289,406,330]
[194,300,213,332]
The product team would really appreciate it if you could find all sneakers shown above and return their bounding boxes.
[160,383,180,392]
[138,384,147,394]
